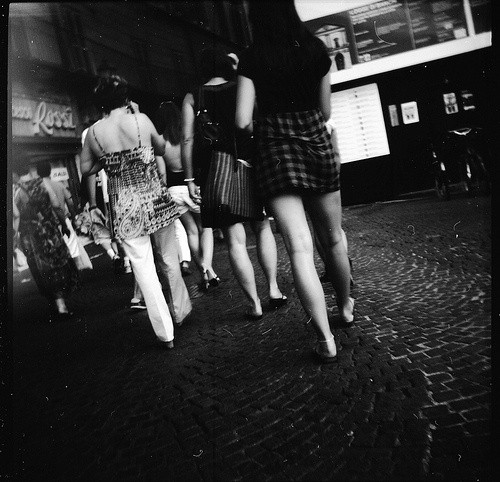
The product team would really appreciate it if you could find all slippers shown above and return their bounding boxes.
[269,295,288,307]
[243,311,263,320]
[341,297,355,327]
[312,344,338,364]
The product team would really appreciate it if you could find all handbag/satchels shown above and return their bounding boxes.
[190,86,238,185]
[200,151,251,229]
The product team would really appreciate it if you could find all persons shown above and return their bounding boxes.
[81,73,220,350]
[183,0,354,359]
[12,159,76,320]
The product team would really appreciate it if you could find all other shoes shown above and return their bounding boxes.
[181,266,194,274]
[130,297,147,309]
[58,310,75,318]
[152,332,174,349]
